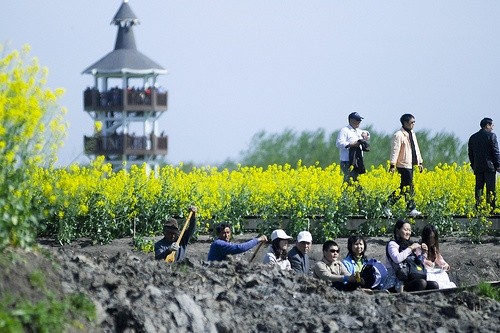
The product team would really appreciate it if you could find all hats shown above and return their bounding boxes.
[270,229,294,241]
[348,111,364,122]
[297,230,312,243]
[164,218,178,230]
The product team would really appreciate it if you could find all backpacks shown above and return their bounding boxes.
[394,239,428,281]
[360,258,394,290]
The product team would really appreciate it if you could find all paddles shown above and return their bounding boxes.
[165,209,192,264]
[250,240,265,262]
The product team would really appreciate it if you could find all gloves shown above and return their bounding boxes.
[168,242,179,252]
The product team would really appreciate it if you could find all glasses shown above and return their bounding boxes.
[330,249,339,253]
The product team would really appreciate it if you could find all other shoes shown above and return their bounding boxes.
[382,207,392,216]
[409,210,422,216]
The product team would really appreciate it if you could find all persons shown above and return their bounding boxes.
[83,128,165,151]
[153,205,198,263]
[206,221,268,262]
[335,111,370,182]
[264,219,456,292]
[468,117,500,209]
[382,114,423,210]
[83,84,159,118]
